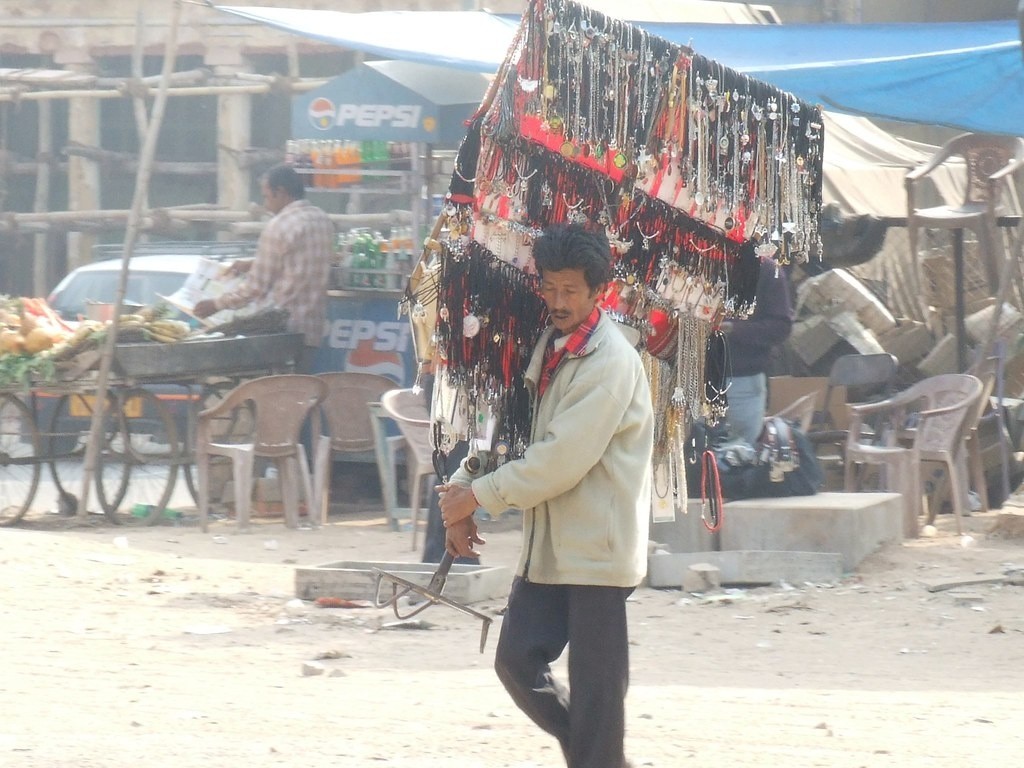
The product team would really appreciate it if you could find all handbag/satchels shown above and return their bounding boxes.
[684,416,820,500]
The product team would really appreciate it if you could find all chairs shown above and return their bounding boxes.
[903,133,1024,301]
[805,354,900,490]
[843,373,984,538]
[197,370,430,551]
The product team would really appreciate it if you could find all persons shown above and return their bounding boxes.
[413,259,481,565]
[719,241,792,446]
[434,219,656,768]
[192,160,339,372]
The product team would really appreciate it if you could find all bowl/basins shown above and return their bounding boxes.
[84,297,146,325]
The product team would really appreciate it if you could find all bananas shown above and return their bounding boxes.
[70,312,189,346]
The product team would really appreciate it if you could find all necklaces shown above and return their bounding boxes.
[426,1,825,502]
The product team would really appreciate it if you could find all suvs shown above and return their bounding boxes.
[34,241,261,454]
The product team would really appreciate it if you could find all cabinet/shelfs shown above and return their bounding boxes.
[284,138,432,305]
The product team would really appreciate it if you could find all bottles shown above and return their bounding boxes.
[282,138,413,189]
[327,225,414,290]
[262,462,279,479]
